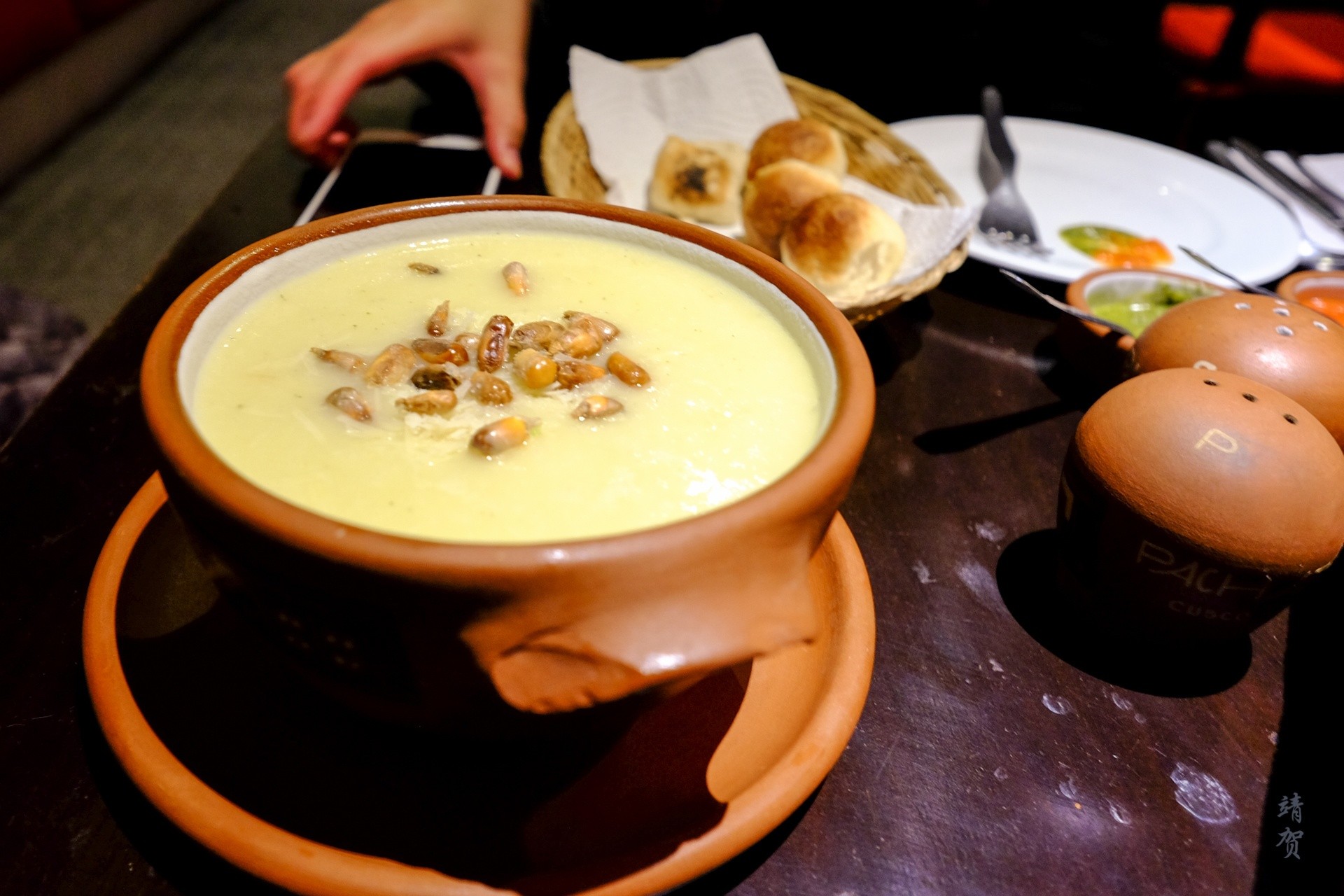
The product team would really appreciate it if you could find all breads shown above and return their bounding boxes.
[650,120,909,302]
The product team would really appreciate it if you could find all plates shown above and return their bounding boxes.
[889,114,1304,289]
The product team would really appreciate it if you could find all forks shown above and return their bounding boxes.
[977,86,1053,255]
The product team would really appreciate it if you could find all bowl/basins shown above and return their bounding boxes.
[1277,270,1344,329]
[140,194,878,726]
[1067,269,1229,351]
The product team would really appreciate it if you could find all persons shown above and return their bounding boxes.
[284,1,1175,176]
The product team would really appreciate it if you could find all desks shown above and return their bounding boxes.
[0,78,1299,896]
